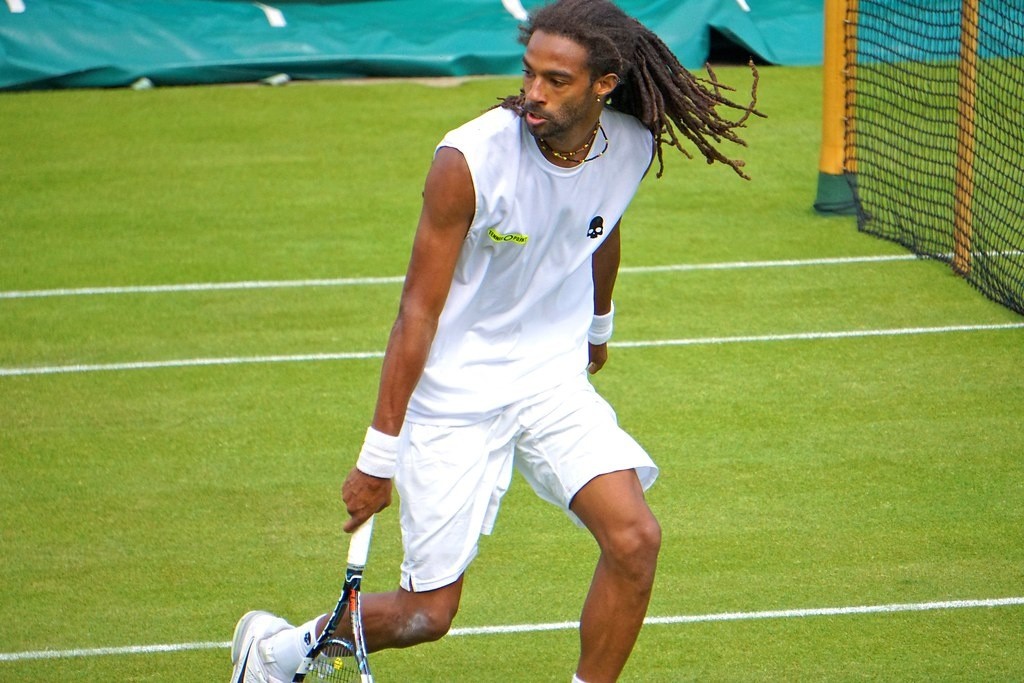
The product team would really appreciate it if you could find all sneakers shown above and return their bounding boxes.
[230,610,295,683]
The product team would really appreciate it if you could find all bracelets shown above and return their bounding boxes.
[356,426,401,478]
[588,300,614,345]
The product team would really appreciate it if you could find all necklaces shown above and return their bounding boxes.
[538,117,610,163]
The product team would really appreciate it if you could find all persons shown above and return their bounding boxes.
[230,0,772,683]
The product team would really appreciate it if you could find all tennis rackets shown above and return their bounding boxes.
[292,506,373,683]
